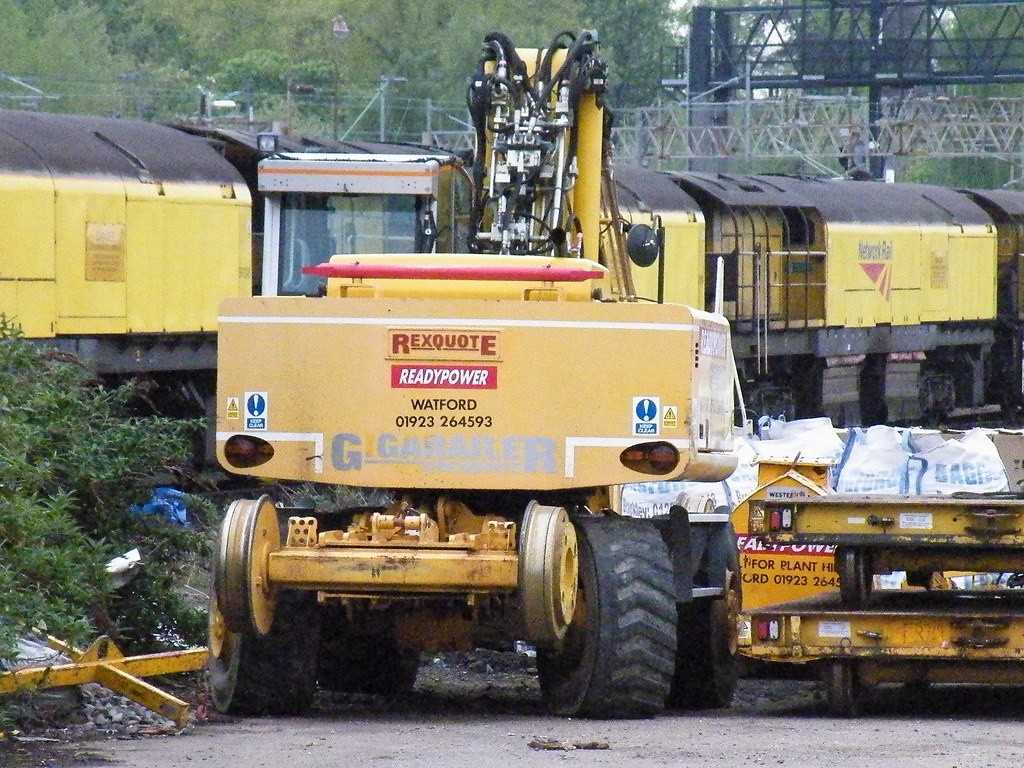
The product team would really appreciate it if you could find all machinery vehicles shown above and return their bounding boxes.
[211,30,741,718]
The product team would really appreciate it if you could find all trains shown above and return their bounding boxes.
[3,102,1024,435]
